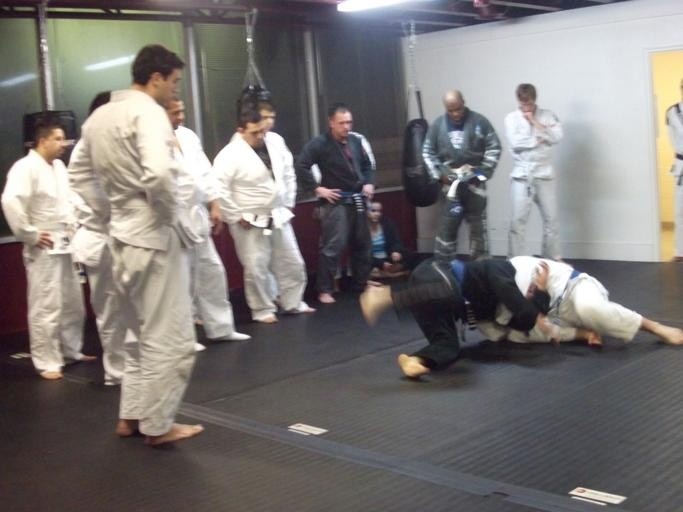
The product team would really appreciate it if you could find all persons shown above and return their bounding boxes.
[362,255,682,376]
[68,42,205,446]
[1,117,98,381]
[211,102,315,323]
[422,89,501,254]
[505,83,561,259]
[294,103,404,303]
[70,90,250,386]
